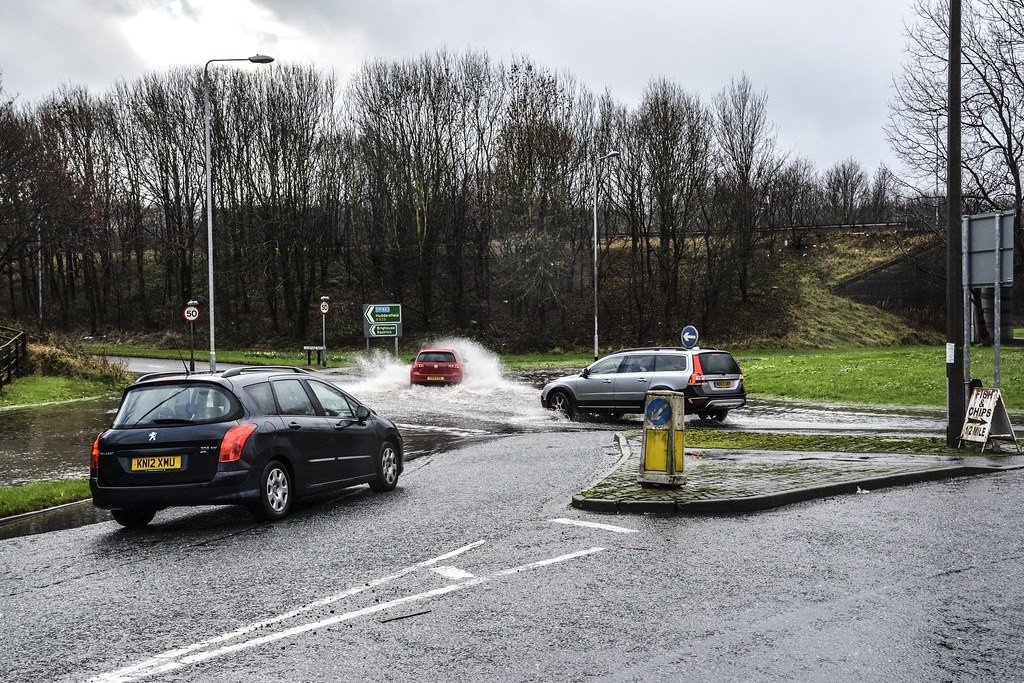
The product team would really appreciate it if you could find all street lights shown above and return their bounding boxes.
[204,54,275,370]
[592,151,621,360]
[936,113,944,230]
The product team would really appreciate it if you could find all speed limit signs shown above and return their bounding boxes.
[319,301,330,315]
[183,306,200,322]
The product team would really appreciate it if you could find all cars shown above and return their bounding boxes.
[410,348,467,386]
[90,363,405,527]
[538,345,745,423]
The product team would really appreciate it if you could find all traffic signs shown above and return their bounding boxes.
[958,387,999,443]
[362,303,401,336]
